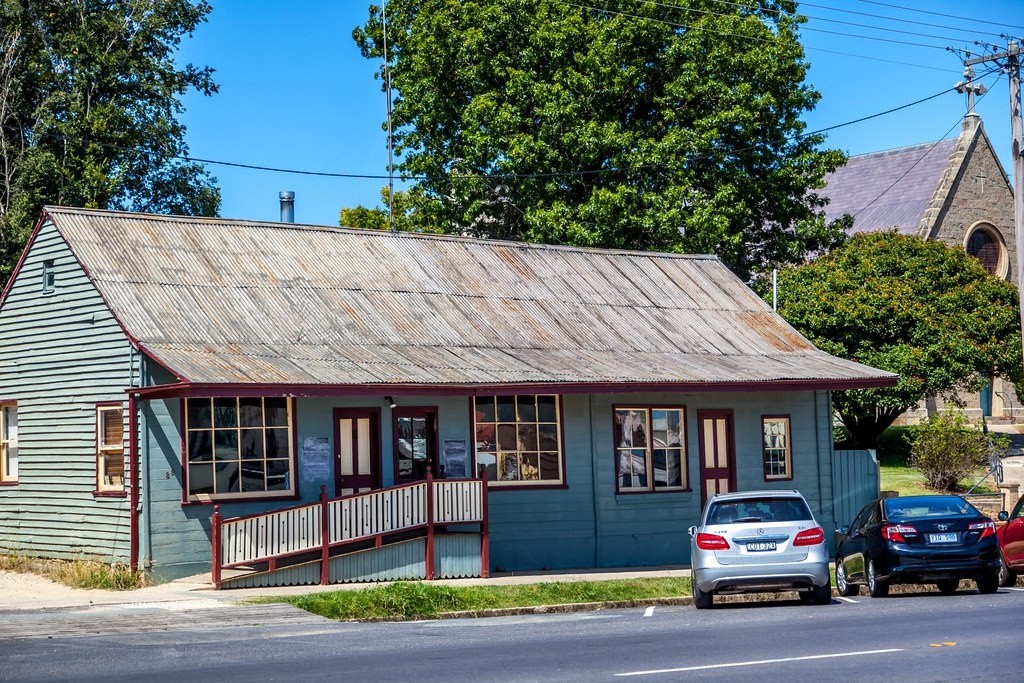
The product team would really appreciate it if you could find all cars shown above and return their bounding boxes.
[994,494,1024,585]
[689,486,832,610]
[837,493,1001,597]
